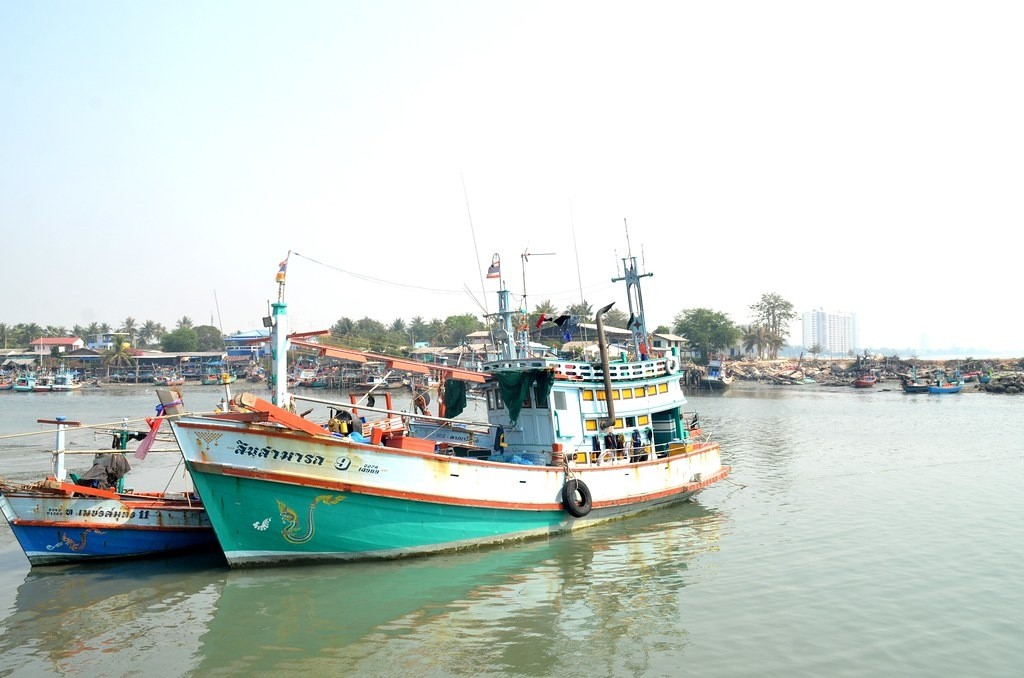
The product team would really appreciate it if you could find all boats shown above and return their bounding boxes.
[0,172,733,571]
[770,348,992,396]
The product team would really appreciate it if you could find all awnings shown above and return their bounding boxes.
[223,354,252,361]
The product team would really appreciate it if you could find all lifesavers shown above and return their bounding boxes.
[666,356,679,374]
[561,478,591,517]
